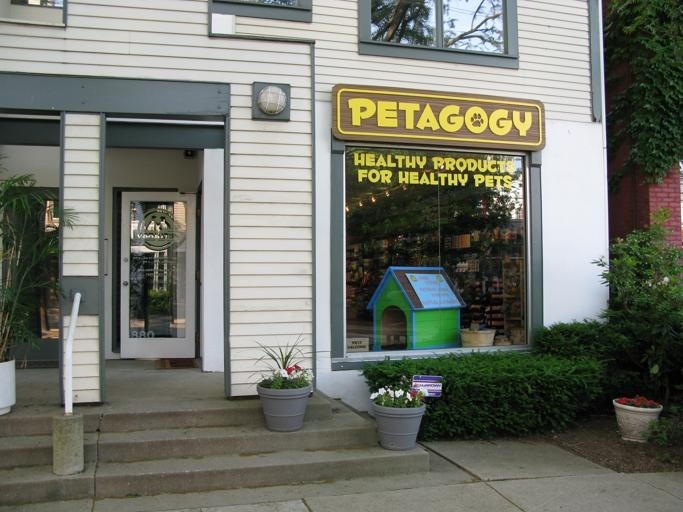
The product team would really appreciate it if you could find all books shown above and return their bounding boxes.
[444,234,470,249]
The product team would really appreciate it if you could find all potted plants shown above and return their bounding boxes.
[0,152,81,418]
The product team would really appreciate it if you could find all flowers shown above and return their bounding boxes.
[240,329,316,391]
[371,360,425,408]
[617,393,661,409]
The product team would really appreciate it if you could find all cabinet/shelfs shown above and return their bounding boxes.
[442,192,524,335]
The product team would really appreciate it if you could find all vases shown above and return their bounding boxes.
[371,401,428,451]
[256,382,312,432]
[612,398,663,444]
